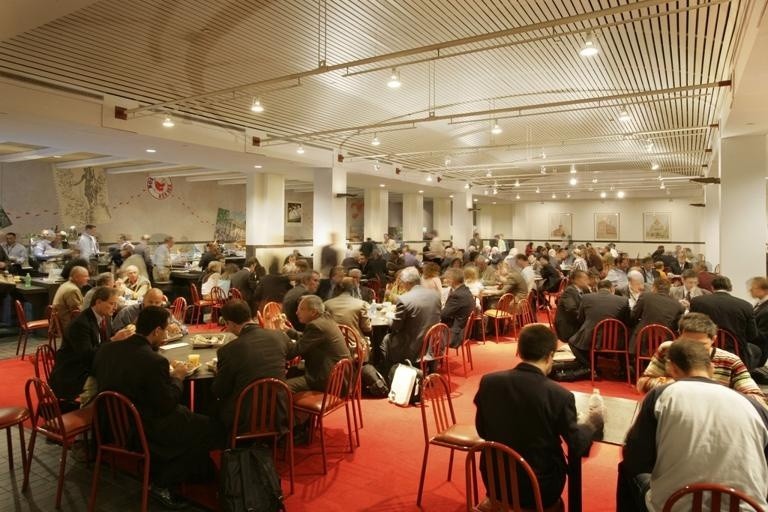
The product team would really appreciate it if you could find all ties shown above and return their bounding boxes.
[685,290,692,305]
[100,318,109,344]
[753,303,760,311]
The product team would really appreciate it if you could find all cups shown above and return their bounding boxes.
[187,353,200,365]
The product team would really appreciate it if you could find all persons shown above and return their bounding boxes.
[46,264,353,511]
[616,313,768,511]
[2,225,225,284]
[554,242,768,375]
[200,228,559,381]
[474,323,599,510]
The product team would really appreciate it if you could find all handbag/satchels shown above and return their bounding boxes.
[549,359,592,381]
[362,359,424,406]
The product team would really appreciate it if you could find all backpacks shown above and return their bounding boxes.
[218,447,286,511]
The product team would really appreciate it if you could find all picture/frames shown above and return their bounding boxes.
[285,199,303,226]
[547,211,574,245]
[592,210,622,243]
[640,210,673,244]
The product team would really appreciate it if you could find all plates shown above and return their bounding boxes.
[191,333,225,348]
[158,333,185,345]
[168,361,202,377]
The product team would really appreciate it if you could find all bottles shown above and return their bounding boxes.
[125,288,143,304]
[370,298,376,317]
[588,388,604,412]
[25,272,31,287]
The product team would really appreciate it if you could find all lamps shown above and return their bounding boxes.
[385,68,402,90]
[161,110,175,128]
[297,122,506,206]
[251,95,266,114]
[615,106,667,193]
[509,153,626,201]
[577,30,600,59]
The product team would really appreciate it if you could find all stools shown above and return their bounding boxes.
[1,401,34,474]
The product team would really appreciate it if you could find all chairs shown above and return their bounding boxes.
[462,440,549,510]
[334,323,374,427]
[285,241,543,392]
[544,250,683,329]
[80,390,164,510]
[414,372,491,508]
[286,357,354,477]
[635,323,677,382]
[586,313,634,384]
[661,482,762,512]
[713,325,741,361]
[227,376,298,500]
[0,257,284,361]
[16,375,96,505]
[27,341,80,450]
